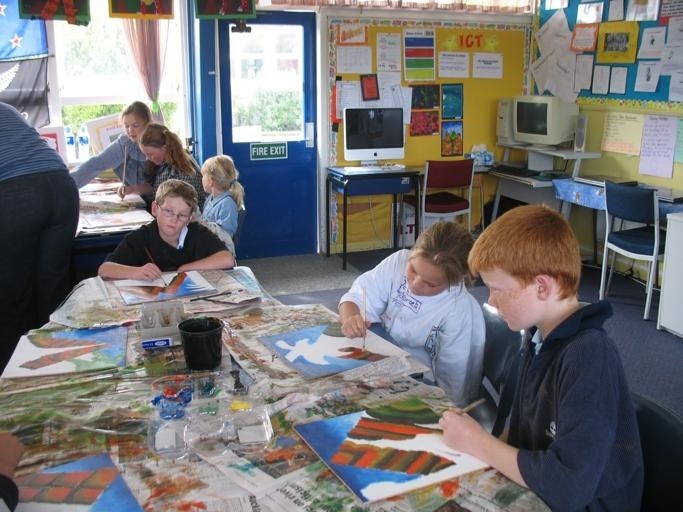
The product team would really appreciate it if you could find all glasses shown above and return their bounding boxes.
[157,204,191,223]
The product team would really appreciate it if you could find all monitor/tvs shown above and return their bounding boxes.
[513,95,579,145]
[344,107,405,164]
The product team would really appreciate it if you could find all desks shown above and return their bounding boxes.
[74,167,155,282]
[0,266,554,512]
[551,175,683,267]
[490,144,601,224]
[327,166,420,269]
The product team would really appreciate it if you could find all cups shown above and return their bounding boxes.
[177,316,226,373]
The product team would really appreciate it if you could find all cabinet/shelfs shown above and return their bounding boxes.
[656,212,683,339]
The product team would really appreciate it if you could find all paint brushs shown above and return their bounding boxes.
[190,288,242,303]
[460,398,487,414]
[115,370,221,382]
[362,286,367,355]
[120,144,127,200]
[144,247,167,286]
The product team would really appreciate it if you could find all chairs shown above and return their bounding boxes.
[473,307,532,438]
[598,179,667,320]
[630,391,683,512]
[397,158,475,235]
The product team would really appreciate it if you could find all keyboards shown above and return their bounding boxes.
[344,165,401,173]
[488,164,541,178]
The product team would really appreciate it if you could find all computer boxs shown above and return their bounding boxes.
[495,100,528,146]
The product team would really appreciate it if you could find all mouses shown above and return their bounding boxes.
[394,164,404,169]
[395,164,406,168]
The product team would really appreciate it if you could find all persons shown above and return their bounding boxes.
[338,220,486,410]
[201,155,245,239]
[0,102,79,374]
[117,123,209,213]
[1,428,26,512]
[69,101,153,202]
[98,179,235,280]
[439,202,644,512]
